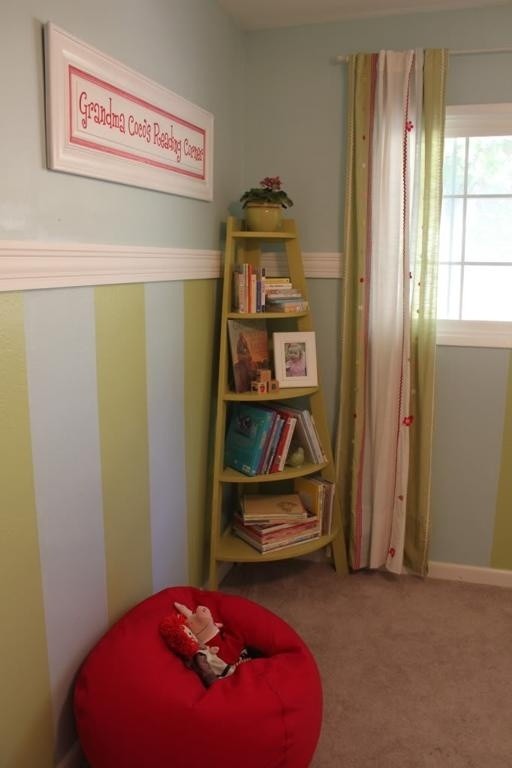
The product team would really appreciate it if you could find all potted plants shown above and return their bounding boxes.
[240,175,294,231]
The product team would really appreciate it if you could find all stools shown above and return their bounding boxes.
[74,587,323,768]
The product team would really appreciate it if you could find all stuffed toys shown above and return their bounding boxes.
[173,602,245,665]
[159,613,237,687]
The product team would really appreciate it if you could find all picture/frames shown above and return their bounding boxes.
[273,331,318,388]
[43,21,214,203]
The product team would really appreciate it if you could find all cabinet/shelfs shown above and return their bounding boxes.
[209,216,349,592]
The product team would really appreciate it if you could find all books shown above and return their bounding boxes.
[226,261,336,555]
